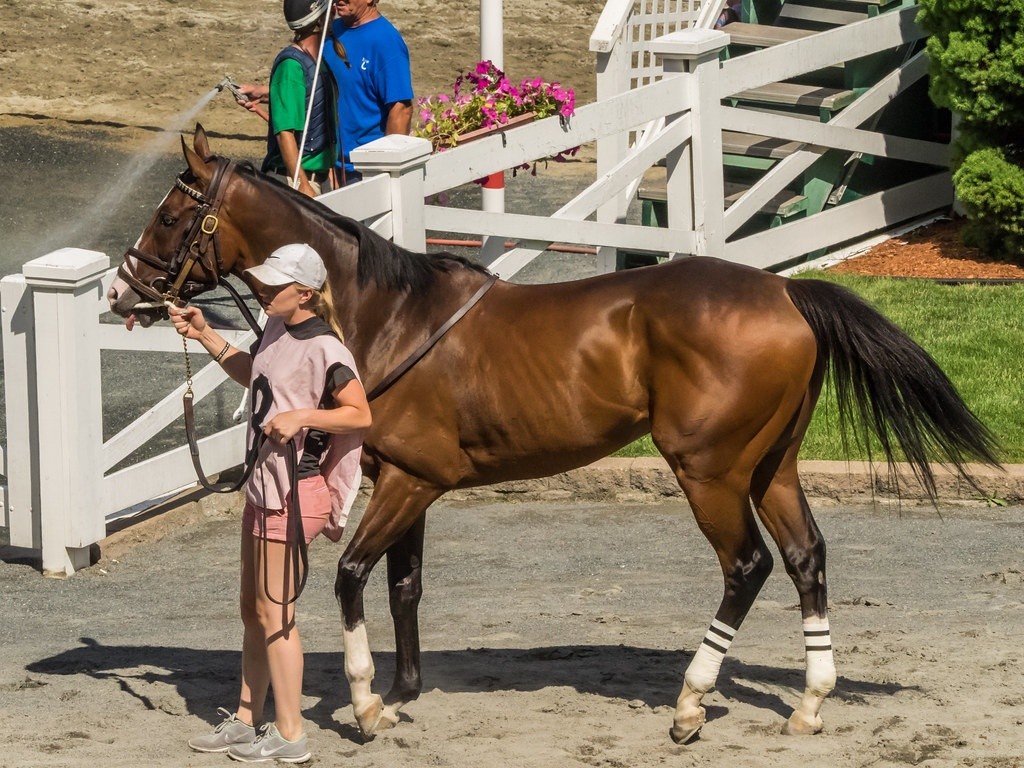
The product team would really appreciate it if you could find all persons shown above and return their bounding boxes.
[236,0,414,198]
[169,244,371,763]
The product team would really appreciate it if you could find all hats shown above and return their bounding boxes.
[242,242,327,290]
[284,0,335,30]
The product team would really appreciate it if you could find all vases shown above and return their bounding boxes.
[438,110,538,149]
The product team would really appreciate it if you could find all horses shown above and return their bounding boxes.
[105,118,1019,752]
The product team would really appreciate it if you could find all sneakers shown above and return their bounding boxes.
[188,707,266,751]
[228,721,312,762]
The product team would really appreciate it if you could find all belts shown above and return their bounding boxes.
[268,165,324,183]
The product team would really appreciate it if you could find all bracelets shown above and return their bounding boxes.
[213,341,231,361]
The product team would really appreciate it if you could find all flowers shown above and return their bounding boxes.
[412,60,582,207]
